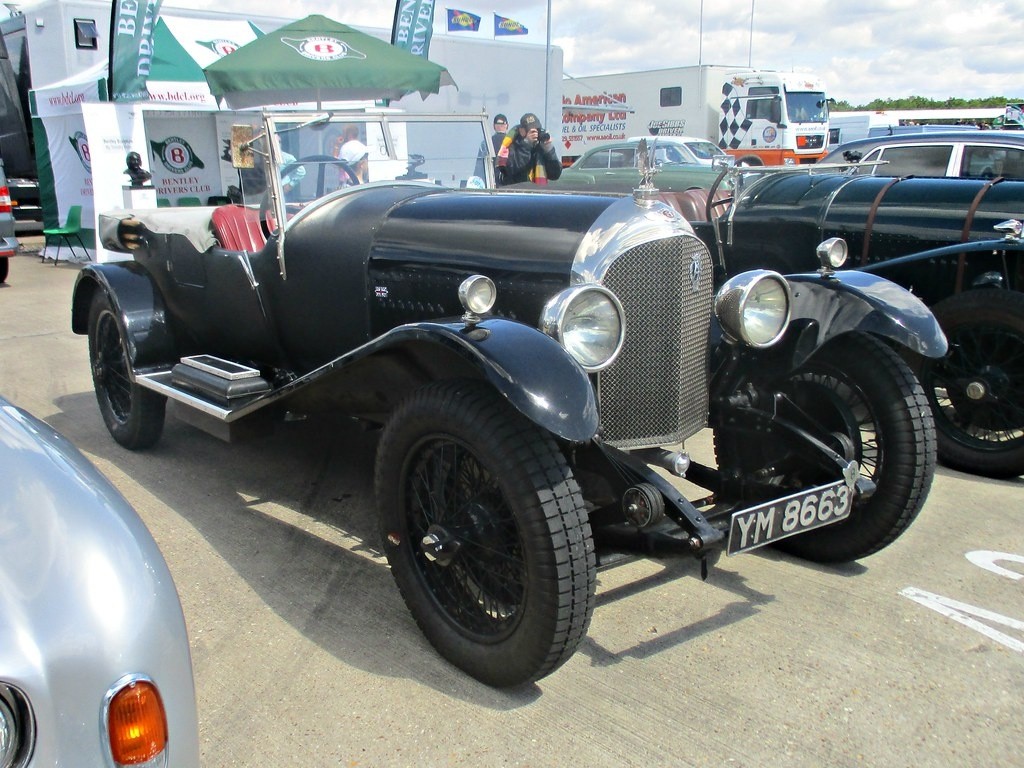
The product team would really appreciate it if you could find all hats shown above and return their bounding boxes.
[493,114,509,130]
[520,113,542,130]
[338,140,376,166]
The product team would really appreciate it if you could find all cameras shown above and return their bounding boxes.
[533,128,550,142]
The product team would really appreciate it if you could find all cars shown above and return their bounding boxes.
[0,155,21,282]
[539,139,741,196]
[625,135,751,180]
[69,111,951,687]
[686,106,1024,476]
[0,392,203,768]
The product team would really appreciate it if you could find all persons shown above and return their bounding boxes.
[274,135,307,204]
[332,125,370,190]
[123,152,152,187]
[473,113,562,189]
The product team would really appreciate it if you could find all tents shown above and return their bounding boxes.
[28,15,388,265]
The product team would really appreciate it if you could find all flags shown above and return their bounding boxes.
[494,14,528,36]
[447,9,481,31]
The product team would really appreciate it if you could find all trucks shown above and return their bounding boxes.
[561,61,835,184]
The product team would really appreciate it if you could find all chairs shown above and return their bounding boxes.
[41,205,92,266]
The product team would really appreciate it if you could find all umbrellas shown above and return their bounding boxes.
[202,16,459,157]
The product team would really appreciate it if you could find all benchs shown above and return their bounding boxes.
[212,200,295,254]
[651,188,737,224]
